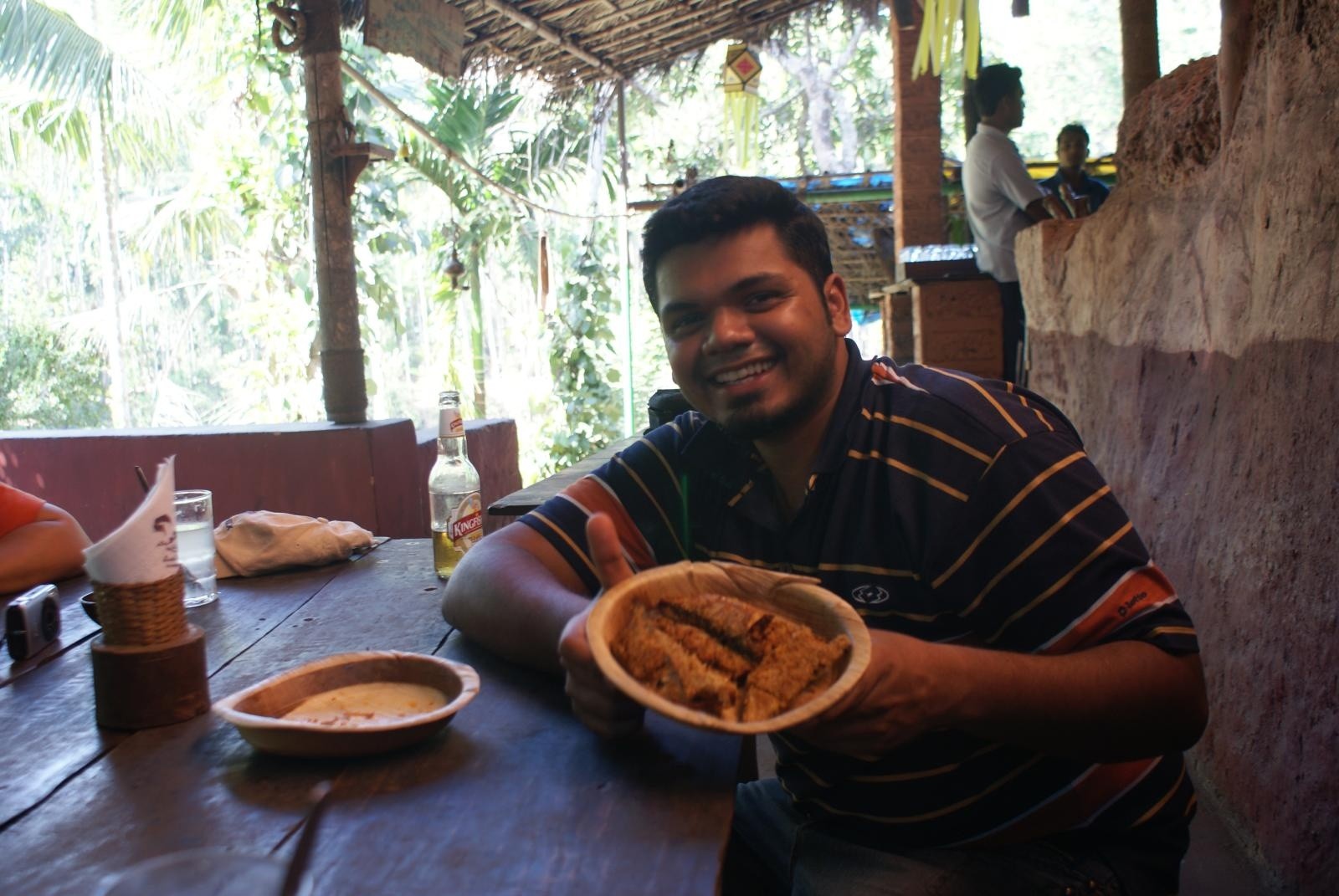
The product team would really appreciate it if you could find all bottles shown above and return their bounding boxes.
[427,390,485,581]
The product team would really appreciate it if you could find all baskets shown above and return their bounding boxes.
[92,560,187,646]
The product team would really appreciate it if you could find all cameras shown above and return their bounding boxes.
[6,584,61,660]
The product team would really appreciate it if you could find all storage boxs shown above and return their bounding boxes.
[868,275,1005,381]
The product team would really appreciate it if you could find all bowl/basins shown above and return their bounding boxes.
[211,650,481,758]
[584,561,871,735]
[78,592,100,625]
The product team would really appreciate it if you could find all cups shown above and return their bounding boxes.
[172,489,217,609]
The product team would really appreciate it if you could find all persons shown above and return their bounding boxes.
[0,482,95,595]
[441,176,1209,896]
[961,62,1111,383]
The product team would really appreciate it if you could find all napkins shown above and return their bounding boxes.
[81,451,180,586]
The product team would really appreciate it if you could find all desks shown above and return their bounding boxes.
[486,426,645,515]
[0,536,759,896]
